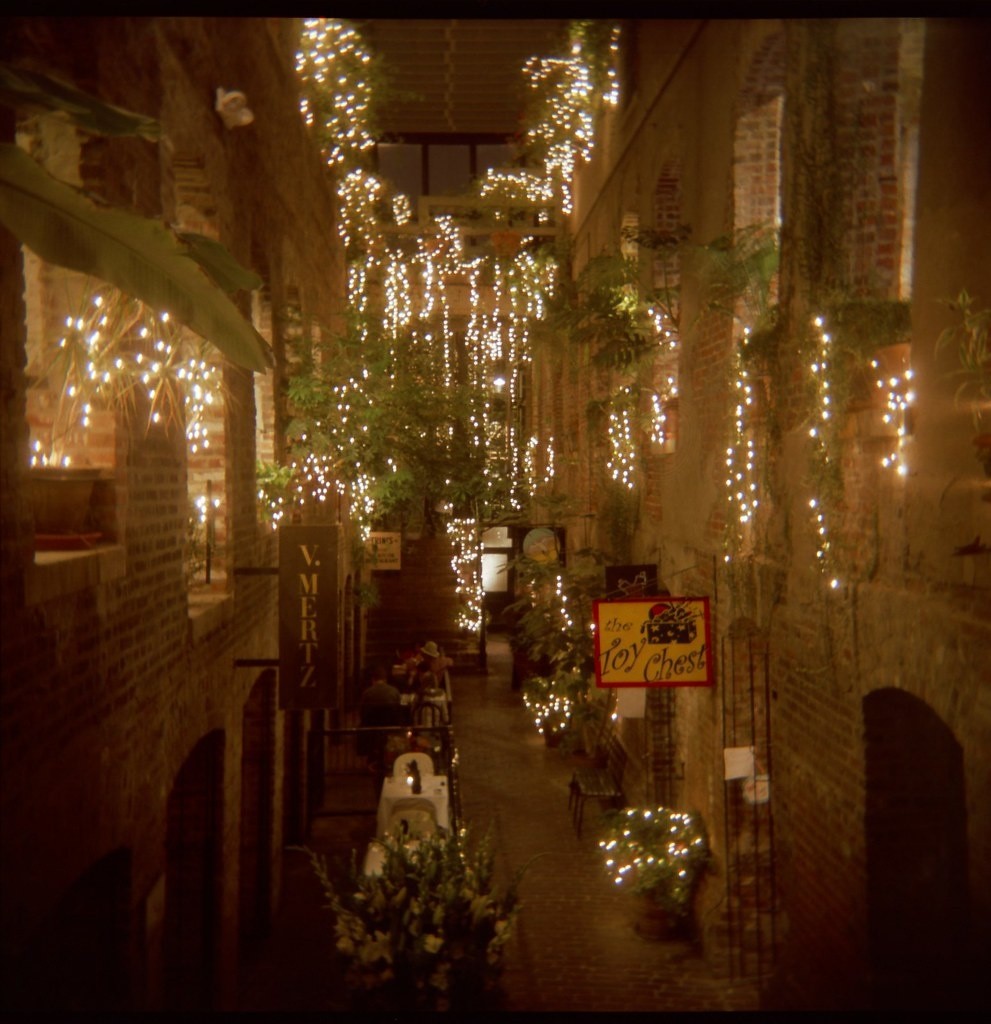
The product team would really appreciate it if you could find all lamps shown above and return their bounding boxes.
[215,86,254,130]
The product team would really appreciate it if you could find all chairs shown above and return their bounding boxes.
[567,738,630,839]
[355,704,414,757]
[393,752,434,776]
[387,810,439,839]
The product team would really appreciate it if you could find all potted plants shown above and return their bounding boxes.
[595,803,712,934]
[523,668,577,749]
[569,687,616,771]
[32,277,237,534]
[405,760,422,794]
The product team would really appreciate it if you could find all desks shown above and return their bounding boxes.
[373,776,450,839]
[401,688,448,726]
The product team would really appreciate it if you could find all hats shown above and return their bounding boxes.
[421,641,439,658]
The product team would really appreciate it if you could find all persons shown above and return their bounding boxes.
[359,669,400,762]
[407,641,444,739]
